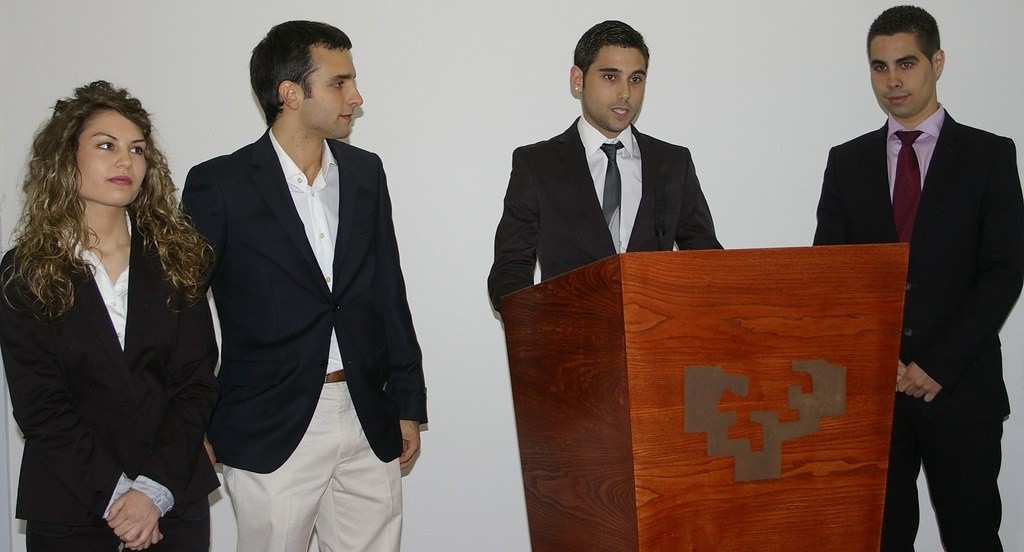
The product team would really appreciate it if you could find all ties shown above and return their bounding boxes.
[600,141,624,254]
[891,131,924,246]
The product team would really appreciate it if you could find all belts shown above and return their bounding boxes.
[323,370,345,383]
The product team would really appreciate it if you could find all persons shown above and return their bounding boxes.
[0,80,222,552]
[487,21,724,312]
[813,5,1024,552]
[179,21,428,552]
